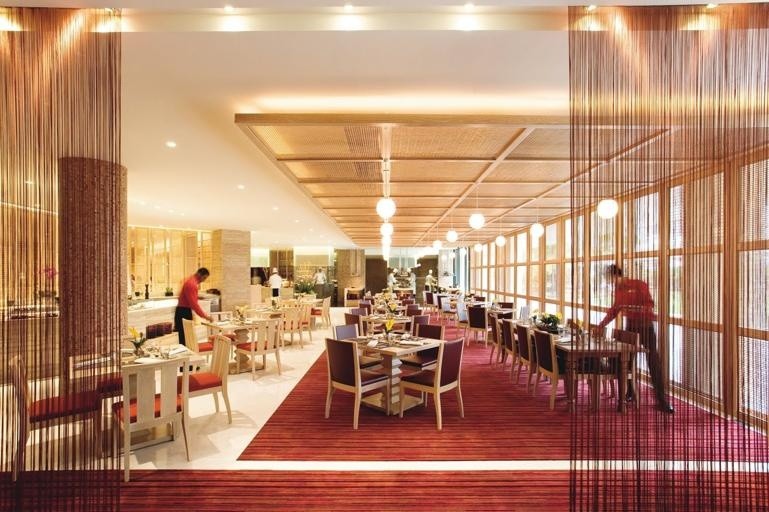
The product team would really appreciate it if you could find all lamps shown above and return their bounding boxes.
[376,182,619,268]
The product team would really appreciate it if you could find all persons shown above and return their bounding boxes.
[313,266,326,285]
[268,267,282,297]
[174,268,216,372]
[386,267,437,295]
[592,263,675,413]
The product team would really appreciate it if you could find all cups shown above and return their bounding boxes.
[5,294,15,307]
[132,321,173,364]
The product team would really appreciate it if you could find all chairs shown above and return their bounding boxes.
[7,289,331,481]
[324,290,649,429]
